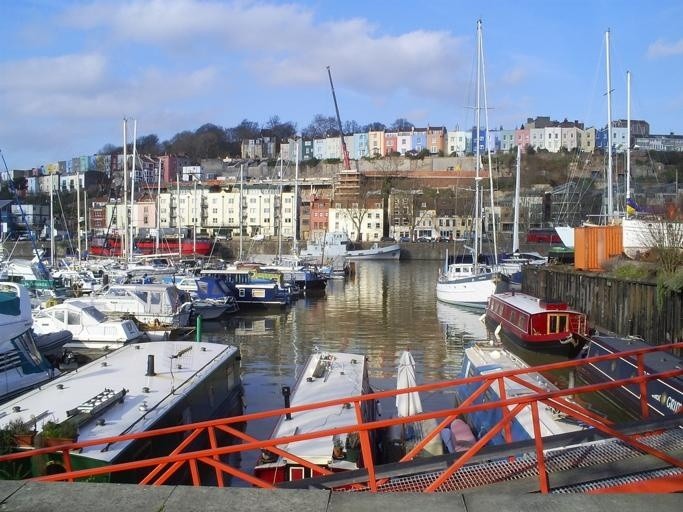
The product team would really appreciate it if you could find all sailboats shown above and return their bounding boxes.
[436,16,681,308]
[0,118,334,349]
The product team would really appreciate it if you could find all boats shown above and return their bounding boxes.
[478,289,682,420]
[341,242,401,260]
[0,342,246,491]
[251,348,379,491]
[453,340,595,467]
